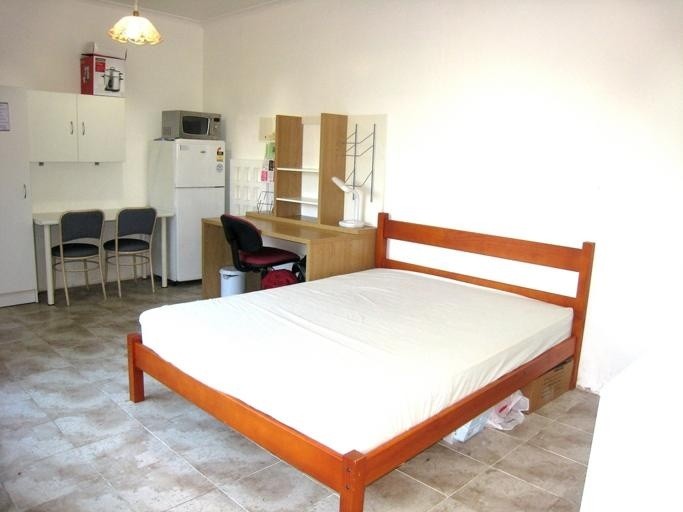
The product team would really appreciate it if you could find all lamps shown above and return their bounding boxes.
[105,0,160,47]
[331,177,366,229]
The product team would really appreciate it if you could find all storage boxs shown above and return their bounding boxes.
[455,407,491,444]
[80,42,129,97]
[518,359,575,415]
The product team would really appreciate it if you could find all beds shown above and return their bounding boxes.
[120,213,596,510]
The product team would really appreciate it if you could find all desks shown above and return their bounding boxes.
[196,212,376,300]
[33,206,175,306]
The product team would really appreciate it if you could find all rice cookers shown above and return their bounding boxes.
[100,67,123,92]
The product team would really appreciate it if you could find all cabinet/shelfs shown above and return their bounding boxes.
[274,113,347,223]
[28,90,126,168]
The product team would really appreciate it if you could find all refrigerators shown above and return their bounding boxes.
[148,139,225,285]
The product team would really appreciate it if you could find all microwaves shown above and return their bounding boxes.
[160,110,221,140]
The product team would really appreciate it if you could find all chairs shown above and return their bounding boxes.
[102,206,157,298]
[222,212,300,278]
[51,207,108,305]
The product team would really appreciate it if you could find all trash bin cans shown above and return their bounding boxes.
[218,265,246,297]
